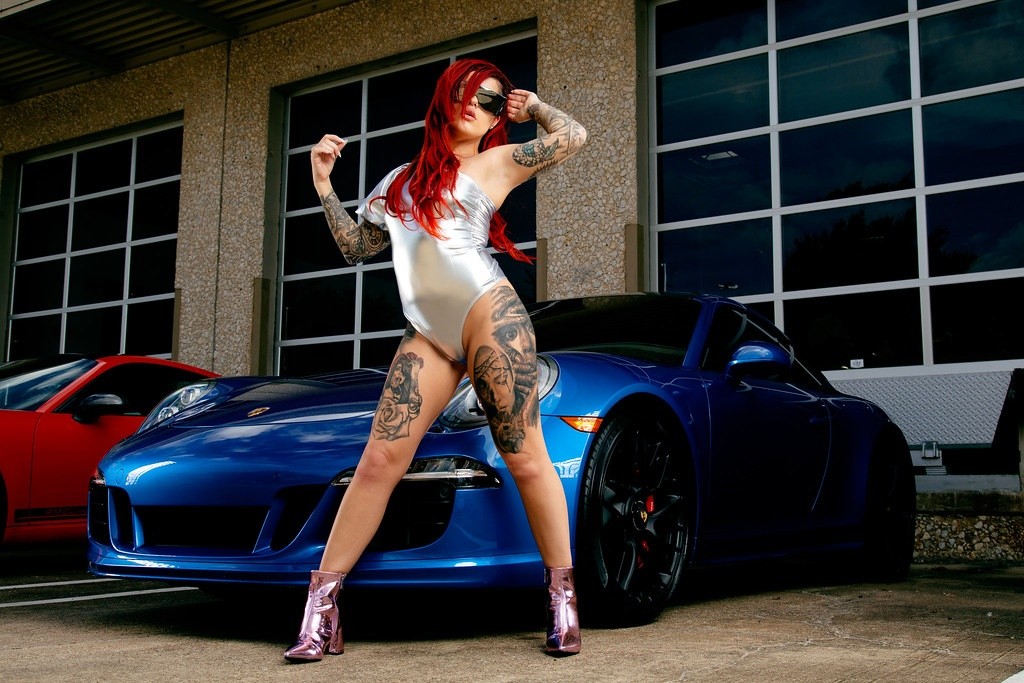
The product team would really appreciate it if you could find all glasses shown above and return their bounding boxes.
[451,82,507,117]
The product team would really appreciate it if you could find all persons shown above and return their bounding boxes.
[284,59,589,662]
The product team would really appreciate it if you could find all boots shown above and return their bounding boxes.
[544,565,582,654]
[283,570,344,661]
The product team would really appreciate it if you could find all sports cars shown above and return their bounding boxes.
[83,286,919,633]
[0,355,225,576]
